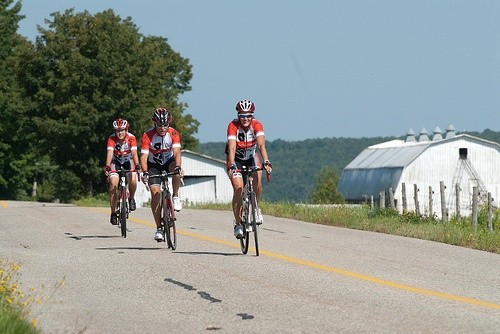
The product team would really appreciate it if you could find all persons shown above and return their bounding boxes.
[225,99,272,239]
[139,107,184,240]
[104,118,141,225]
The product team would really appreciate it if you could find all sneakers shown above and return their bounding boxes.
[153,232,163,240]
[111,213,117,224]
[234,224,243,236]
[255,208,263,224]
[129,197,136,211]
[171,194,182,211]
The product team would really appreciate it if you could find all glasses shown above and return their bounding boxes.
[239,114,252,118]
[157,124,168,127]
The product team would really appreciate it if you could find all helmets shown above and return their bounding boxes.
[152,108,171,123]
[236,99,255,113]
[113,118,128,130]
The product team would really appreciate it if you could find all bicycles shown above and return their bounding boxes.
[141,168,184,250]
[229,168,271,257]
[106,166,142,238]
